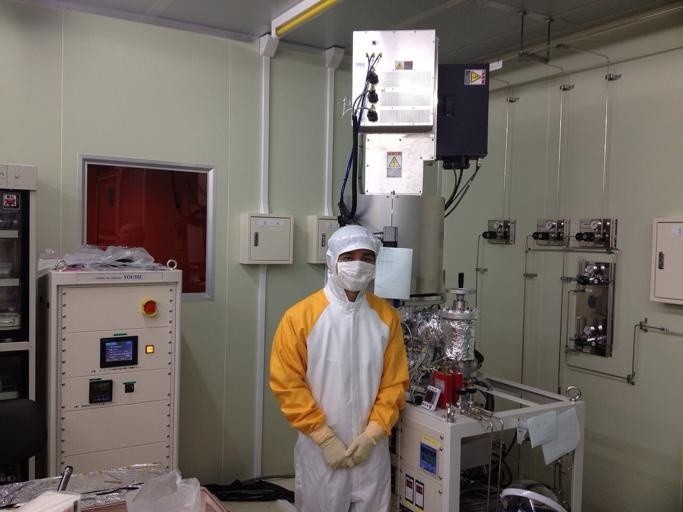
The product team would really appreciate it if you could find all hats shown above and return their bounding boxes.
[326,225,381,277]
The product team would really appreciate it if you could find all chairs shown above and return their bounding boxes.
[0,398,46,481]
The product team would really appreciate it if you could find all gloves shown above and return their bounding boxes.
[344,432,377,467]
[318,431,355,469]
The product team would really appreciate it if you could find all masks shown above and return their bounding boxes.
[338,260,375,294]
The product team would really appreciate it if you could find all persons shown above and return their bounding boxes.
[269,222,409,511]
